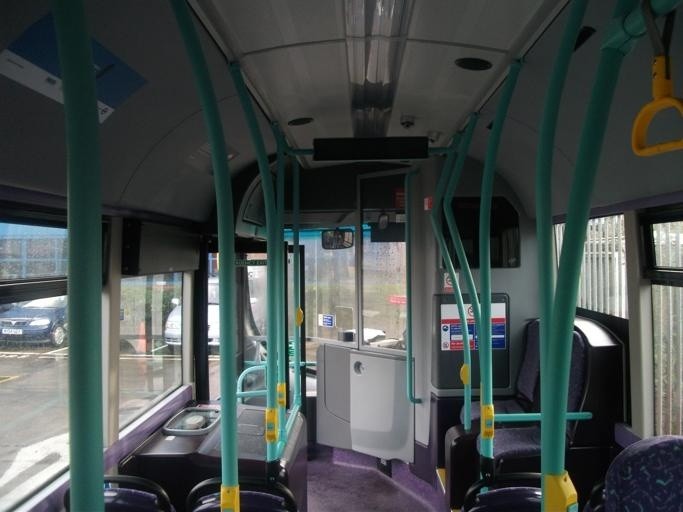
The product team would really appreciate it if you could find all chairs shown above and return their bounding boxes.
[445,315,683,512]
[63,475,297,511]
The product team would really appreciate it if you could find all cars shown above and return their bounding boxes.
[0,294,71,348]
[162,272,259,352]
